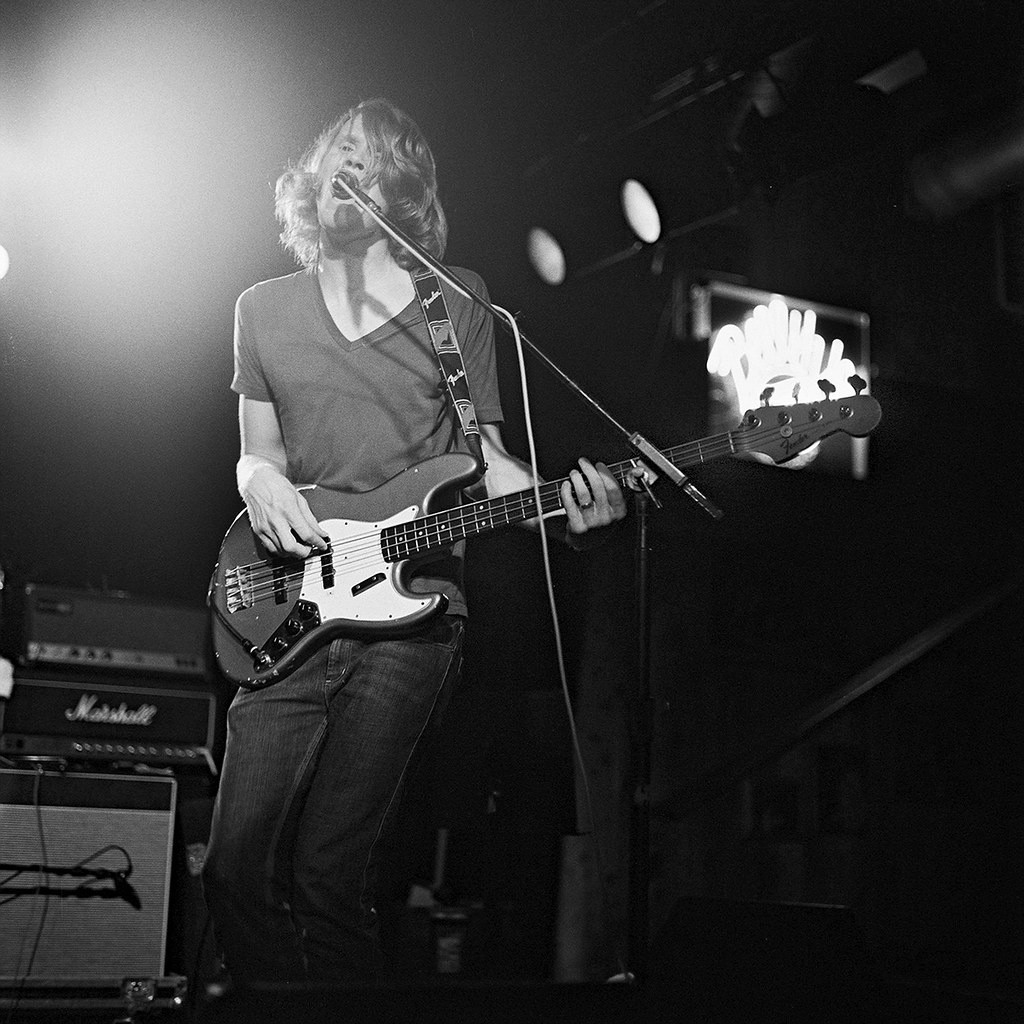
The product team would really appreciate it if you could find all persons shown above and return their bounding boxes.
[200,98,629,987]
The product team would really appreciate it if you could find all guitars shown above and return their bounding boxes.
[205,373,883,691]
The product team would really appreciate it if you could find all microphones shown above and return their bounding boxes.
[111,870,142,910]
[329,170,361,199]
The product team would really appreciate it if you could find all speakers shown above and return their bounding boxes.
[2,771,183,992]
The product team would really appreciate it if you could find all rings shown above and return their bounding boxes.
[582,500,594,509]
